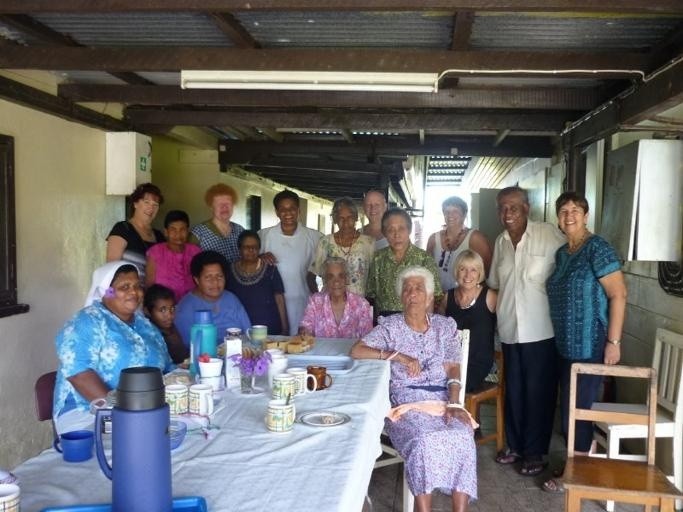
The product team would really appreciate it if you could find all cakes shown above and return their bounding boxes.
[279,340,288,354]
[288,334,313,355]
[266,339,278,350]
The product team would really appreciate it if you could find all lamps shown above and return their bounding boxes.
[180,71,438,94]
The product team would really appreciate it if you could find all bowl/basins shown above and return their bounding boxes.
[168,421,187,450]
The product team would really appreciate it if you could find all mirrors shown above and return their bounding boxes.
[0,134,29,319]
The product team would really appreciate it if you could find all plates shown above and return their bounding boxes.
[285,354,358,374]
[296,410,351,428]
[106,394,227,418]
[40,496,207,512]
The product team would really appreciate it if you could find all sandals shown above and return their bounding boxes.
[495,448,519,464]
[519,456,549,477]
[542,477,564,494]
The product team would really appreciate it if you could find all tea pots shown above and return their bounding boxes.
[190,310,217,376]
[95,367,173,512]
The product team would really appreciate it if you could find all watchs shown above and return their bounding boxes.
[606,338,621,345]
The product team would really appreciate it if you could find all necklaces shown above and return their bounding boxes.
[455,288,477,309]
[443,229,464,273]
[339,230,355,261]
[130,219,154,241]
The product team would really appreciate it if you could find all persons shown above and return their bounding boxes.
[257,189,326,337]
[544,191,628,494]
[187,183,246,264]
[349,265,480,512]
[486,187,570,478]
[296,256,374,337]
[52,260,191,438]
[426,196,493,292]
[170,251,252,354]
[365,208,444,314]
[145,210,205,306]
[358,188,390,252]
[105,182,172,275]
[436,248,497,441]
[144,283,189,364]
[223,230,290,336]
[307,197,377,299]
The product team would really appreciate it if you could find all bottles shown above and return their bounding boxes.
[223,327,242,356]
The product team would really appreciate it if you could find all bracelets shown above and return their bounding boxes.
[380,348,384,359]
[445,401,462,408]
[446,378,461,392]
[568,230,586,252]
[385,350,400,361]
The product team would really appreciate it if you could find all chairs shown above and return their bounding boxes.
[588,327,683,511]
[560,363,683,512]
[34,371,57,440]
[372,328,469,512]
[465,350,505,453]
[369,305,372,319]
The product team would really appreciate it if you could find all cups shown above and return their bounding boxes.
[307,365,332,391]
[226,327,242,338]
[268,355,287,389]
[54,430,94,463]
[166,385,189,415]
[246,325,267,344]
[264,349,283,360]
[0,483,21,512]
[265,399,296,432]
[190,384,214,416]
[271,374,302,401]
[198,357,223,390]
[286,367,318,397]
